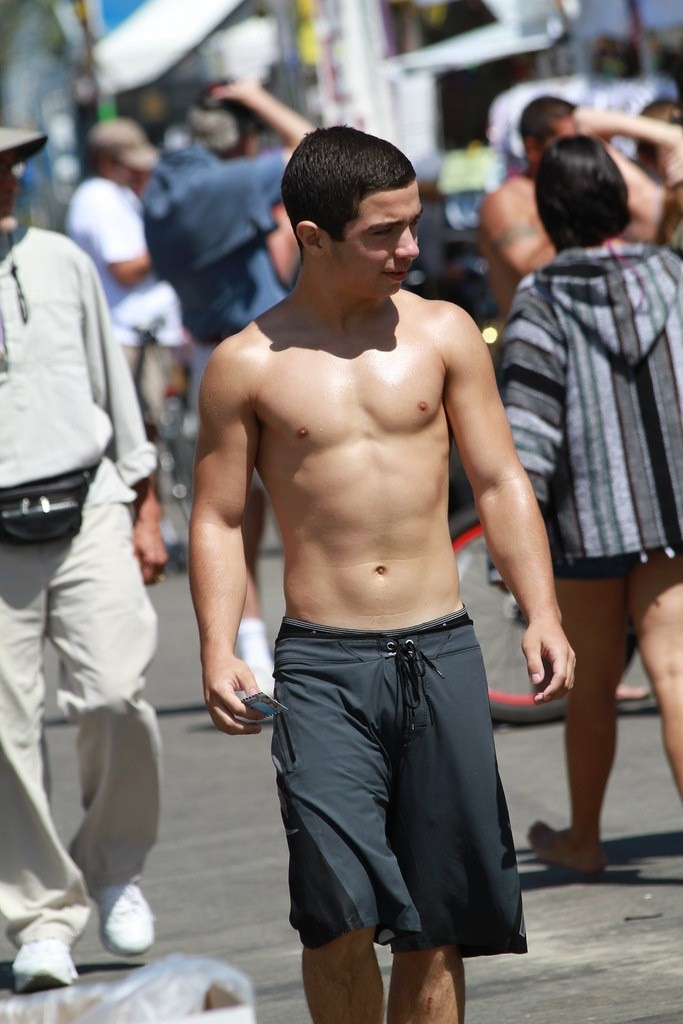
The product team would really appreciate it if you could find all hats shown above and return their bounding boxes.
[88,115,160,172]
[0,123,47,167]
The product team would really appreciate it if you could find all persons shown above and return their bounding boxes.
[64,76,322,723]
[188,125,576,1024]
[474,96,683,878]
[0,128,169,993]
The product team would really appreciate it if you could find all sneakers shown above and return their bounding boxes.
[11,940,75,994]
[86,872,155,955]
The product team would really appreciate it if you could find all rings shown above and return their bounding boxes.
[156,574,166,582]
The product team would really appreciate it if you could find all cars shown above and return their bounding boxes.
[405,152,511,318]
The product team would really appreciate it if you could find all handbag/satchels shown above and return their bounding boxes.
[0,461,102,544]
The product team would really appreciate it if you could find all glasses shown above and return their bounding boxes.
[0,162,26,181]
